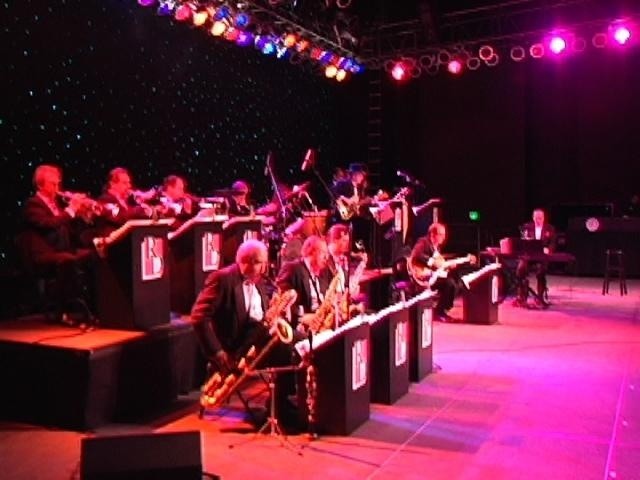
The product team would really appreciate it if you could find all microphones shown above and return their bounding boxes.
[263,152,272,177]
[396,171,414,183]
[301,146,312,170]
[283,181,312,201]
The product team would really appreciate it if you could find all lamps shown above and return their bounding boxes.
[139,0,364,85]
[366,22,640,86]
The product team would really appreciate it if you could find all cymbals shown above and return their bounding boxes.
[262,215,277,226]
[283,181,308,202]
[254,202,279,216]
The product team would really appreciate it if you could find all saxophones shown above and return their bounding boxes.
[296,262,345,338]
[341,251,368,320]
[198,289,297,412]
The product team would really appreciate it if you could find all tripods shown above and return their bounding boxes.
[227,374,314,456]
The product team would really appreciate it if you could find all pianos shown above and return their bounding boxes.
[479,247,577,262]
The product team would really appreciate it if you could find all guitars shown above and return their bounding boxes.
[408,253,473,287]
[337,192,388,221]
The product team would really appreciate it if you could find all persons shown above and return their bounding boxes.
[370,224,405,268]
[330,162,390,253]
[225,180,256,217]
[152,175,193,225]
[513,209,556,309]
[88,167,152,242]
[322,223,367,313]
[272,235,343,341]
[407,223,458,323]
[391,174,420,218]
[191,239,302,431]
[16,164,93,325]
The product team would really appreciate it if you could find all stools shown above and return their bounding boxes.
[602,249,628,297]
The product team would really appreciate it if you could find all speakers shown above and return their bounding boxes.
[80,429,203,480]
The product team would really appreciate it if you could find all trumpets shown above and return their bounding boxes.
[55,190,119,225]
[179,192,229,215]
[127,186,183,216]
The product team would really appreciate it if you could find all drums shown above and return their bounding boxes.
[303,211,329,238]
[284,218,304,239]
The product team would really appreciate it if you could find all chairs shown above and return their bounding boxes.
[193,326,272,425]
